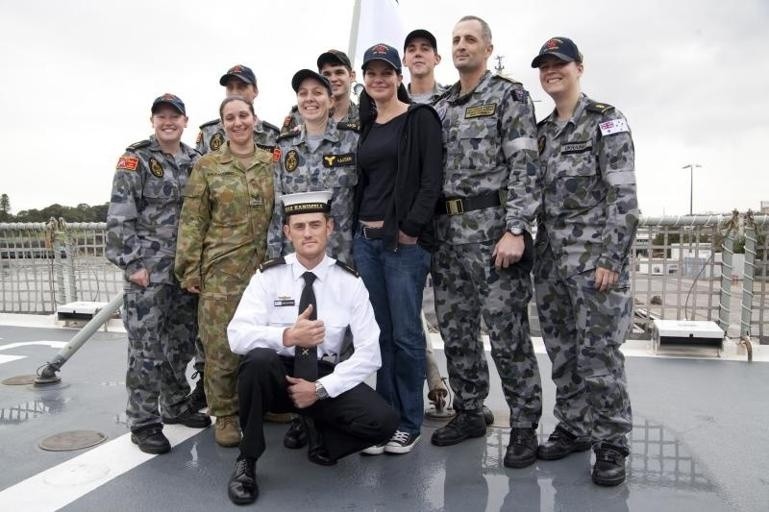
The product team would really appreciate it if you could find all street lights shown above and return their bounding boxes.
[680,164,701,217]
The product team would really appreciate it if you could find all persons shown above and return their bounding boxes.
[280,48,361,138]
[173,95,289,450]
[351,43,443,457]
[401,28,495,425]
[187,63,280,411]
[259,68,362,450]
[530,35,639,489]
[104,92,213,456]
[225,188,402,506]
[430,14,544,472]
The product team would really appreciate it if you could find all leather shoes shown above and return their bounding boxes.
[300,414,343,467]
[163,409,211,429]
[130,426,172,455]
[227,456,259,504]
[283,417,309,450]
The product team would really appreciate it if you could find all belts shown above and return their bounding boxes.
[355,222,386,241]
[433,192,502,216]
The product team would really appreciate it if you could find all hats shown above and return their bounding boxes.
[531,37,581,68]
[316,49,352,68]
[404,29,437,50]
[279,190,334,216]
[361,44,402,68]
[220,65,256,85]
[151,94,185,114]
[291,70,333,98]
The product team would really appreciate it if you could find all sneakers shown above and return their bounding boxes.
[432,410,491,446]
[361,441,384,457]
[593,442,627,486]
[383,428,422,455]
[503,428,538,469]
[214,416,243,448]
[538,425,591,460]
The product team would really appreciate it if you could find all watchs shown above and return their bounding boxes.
[506,224,523,236]
[315,381,328,401]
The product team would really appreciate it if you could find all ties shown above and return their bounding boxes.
[294,270,319,383]
